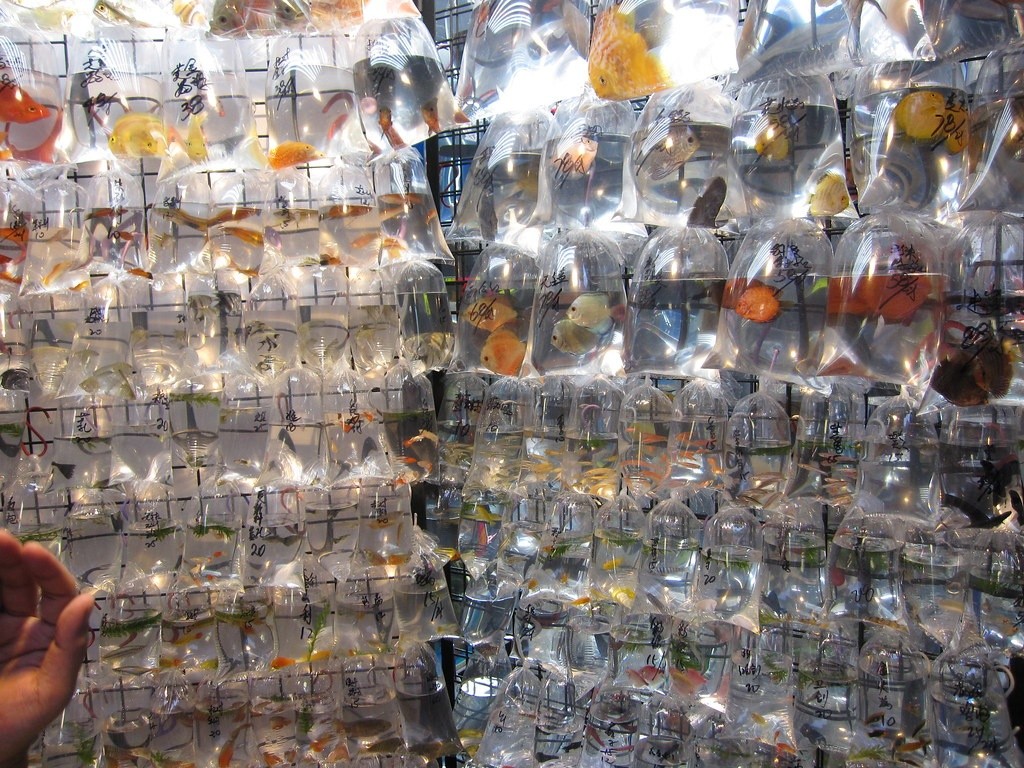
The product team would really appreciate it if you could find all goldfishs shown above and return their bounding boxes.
[2,0,1024,768]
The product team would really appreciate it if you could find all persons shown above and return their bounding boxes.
[0,529,96,766]
